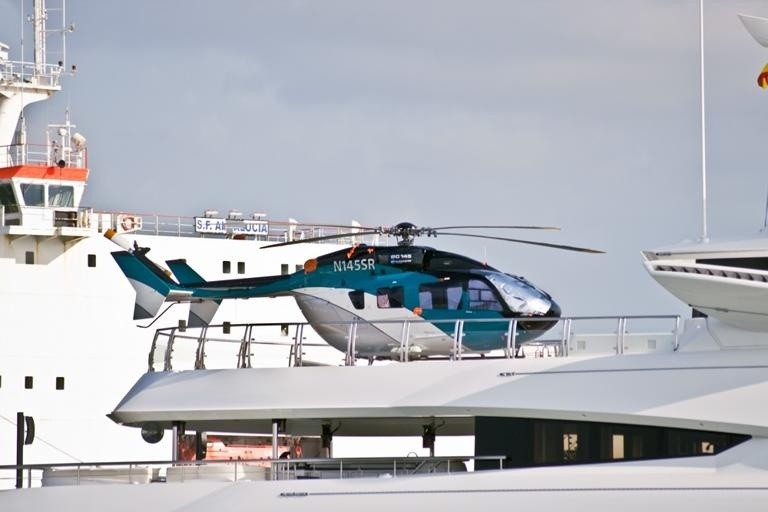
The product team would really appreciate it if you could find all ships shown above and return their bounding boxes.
[1,1,475,487]
[91,214,604,363]
[1,15,768,512]
